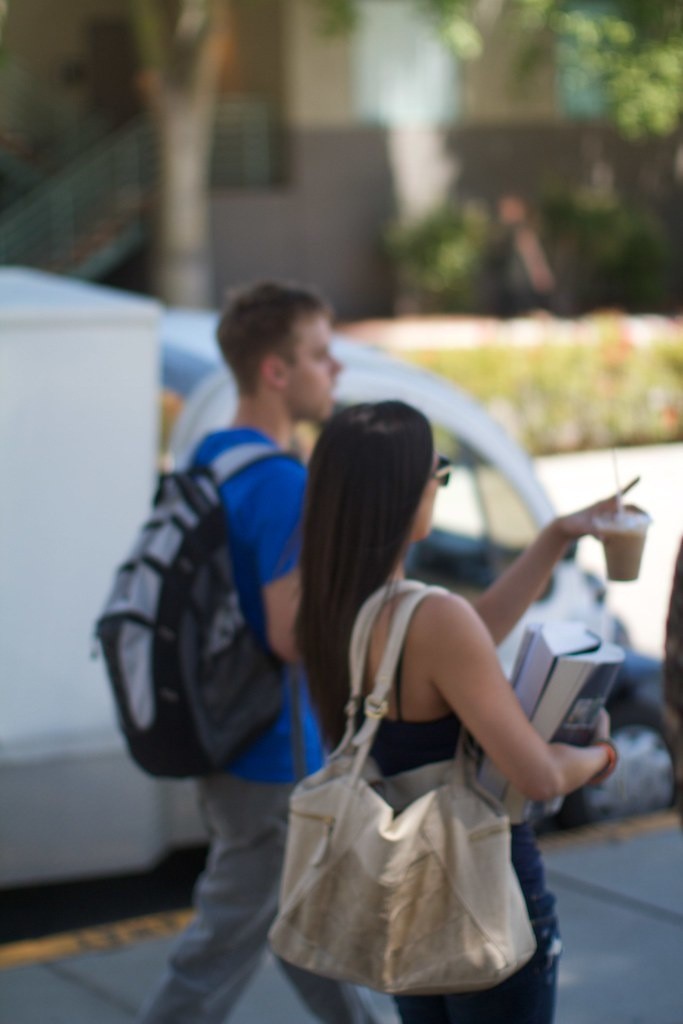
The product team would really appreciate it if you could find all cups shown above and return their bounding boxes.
[593,509,651,580]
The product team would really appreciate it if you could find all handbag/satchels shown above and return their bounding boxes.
[269,580,540,997]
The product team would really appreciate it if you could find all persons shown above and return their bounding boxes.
[142,282,385,1024]
[494,195,556,319]
[662,535,683,833]
[298,400,645,1024]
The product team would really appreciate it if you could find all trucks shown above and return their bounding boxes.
[0,263,605,888]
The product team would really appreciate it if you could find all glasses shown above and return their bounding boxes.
[428,455,453,488]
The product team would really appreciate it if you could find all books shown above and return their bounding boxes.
[479,617,625,824]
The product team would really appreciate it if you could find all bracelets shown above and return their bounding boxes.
[587,736,617,786]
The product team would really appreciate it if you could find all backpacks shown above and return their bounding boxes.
[97,443,307,778]
